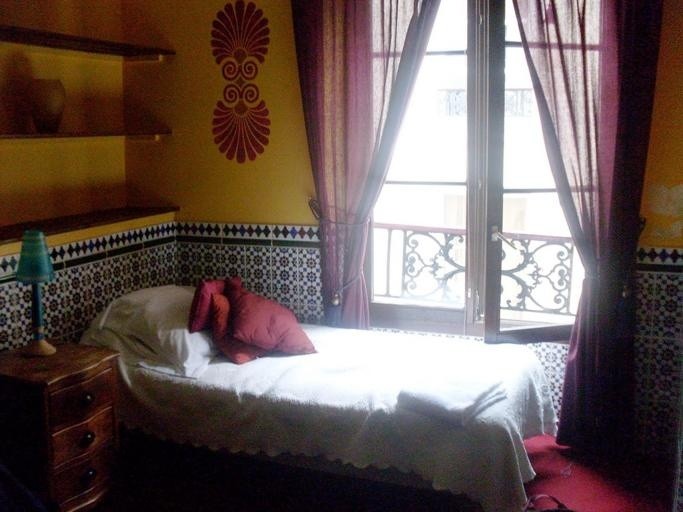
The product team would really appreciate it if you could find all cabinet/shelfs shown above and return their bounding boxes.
[0,25,174,147]
[0,338,121,512]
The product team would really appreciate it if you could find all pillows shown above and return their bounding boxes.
[94,276,318,379]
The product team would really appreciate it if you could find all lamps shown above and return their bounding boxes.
[15,230,58,356]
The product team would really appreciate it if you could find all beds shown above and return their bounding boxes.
[80,282,561,512]
